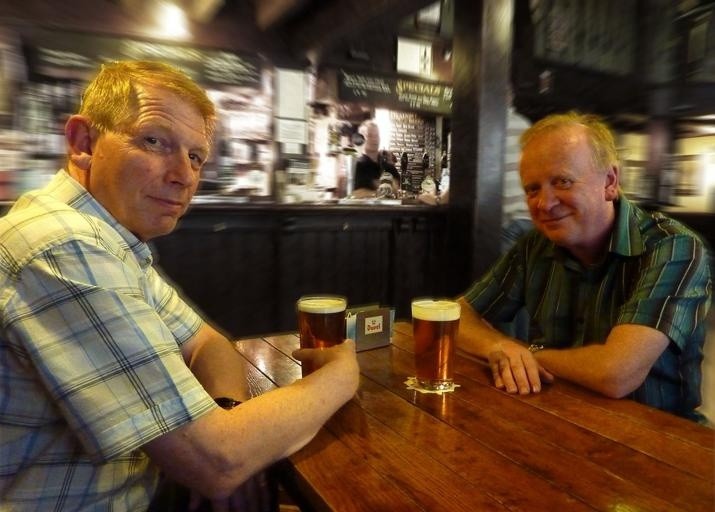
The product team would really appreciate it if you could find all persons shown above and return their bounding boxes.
[347,120,403,202]
[448,109,715,429]
[416,78,535,343]
[0,57,360,511]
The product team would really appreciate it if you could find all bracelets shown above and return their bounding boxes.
[435,195,442,207]
[525,342,545,354]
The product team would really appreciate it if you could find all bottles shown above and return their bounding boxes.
[270,139,288,204]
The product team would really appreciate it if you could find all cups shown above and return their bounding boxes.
[295,299,347,376]
[409,299,462,388]
